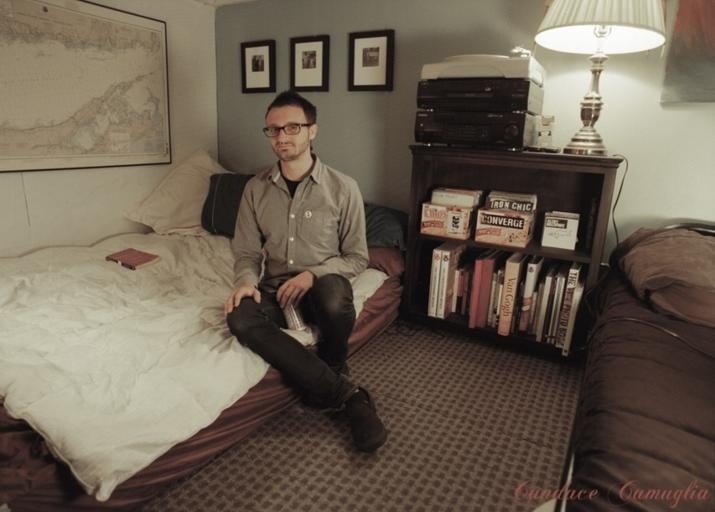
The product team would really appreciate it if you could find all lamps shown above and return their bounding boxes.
[534,0,666,157]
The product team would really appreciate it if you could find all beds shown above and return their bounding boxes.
[0,210,417,512]
[551,222,715,512]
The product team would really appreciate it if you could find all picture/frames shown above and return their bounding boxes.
[288,34,330,92]
[240,38,276,94]
[0,0,173,173]
[347,29,395,91]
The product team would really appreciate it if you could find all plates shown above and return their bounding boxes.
[528,145,561,152]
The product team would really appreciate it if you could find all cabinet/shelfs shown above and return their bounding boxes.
[398,144,624,363]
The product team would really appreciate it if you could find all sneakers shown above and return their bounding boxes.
[344,387,387,453]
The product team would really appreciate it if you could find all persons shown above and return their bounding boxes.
[228,90,394,453]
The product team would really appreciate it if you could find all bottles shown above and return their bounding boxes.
[282,304,305,331]
[539,116,553,146]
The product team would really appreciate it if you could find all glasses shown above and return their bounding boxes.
[262,123,310,137]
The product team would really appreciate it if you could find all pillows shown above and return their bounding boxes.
[123,146,408,275]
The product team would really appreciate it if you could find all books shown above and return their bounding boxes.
[104,245,161,272]
[421,184,583,360]
